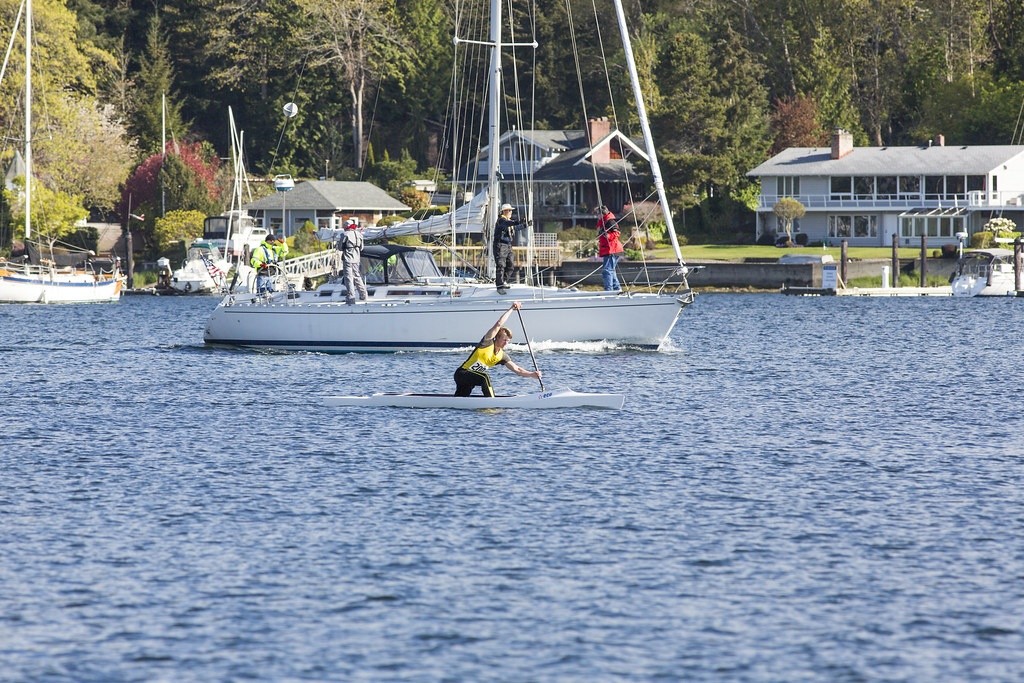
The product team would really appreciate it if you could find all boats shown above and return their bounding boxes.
[167,238,233,296]
[948,247,1023,297]
[306,387,628,411]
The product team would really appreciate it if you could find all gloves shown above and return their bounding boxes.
[528,220,532,225]
[520,218,525,224]
[260,263,266,269]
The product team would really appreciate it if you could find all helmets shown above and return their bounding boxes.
[499,204,515,212]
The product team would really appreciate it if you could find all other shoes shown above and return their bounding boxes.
[503,286,510,289]
[497,289,507,295]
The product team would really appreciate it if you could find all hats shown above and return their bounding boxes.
[265,234,275,241]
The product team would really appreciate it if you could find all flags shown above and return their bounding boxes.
[201,256,223,277]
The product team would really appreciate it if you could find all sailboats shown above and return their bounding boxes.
[201,1,708,350]
[1,0,125,304]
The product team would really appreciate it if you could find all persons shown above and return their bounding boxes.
[337,219,368,304]
[493,203,532,295]
[369,238,395,272]
[454,302,541,398]
[250,233,288,294]
[592,205,623,290]
